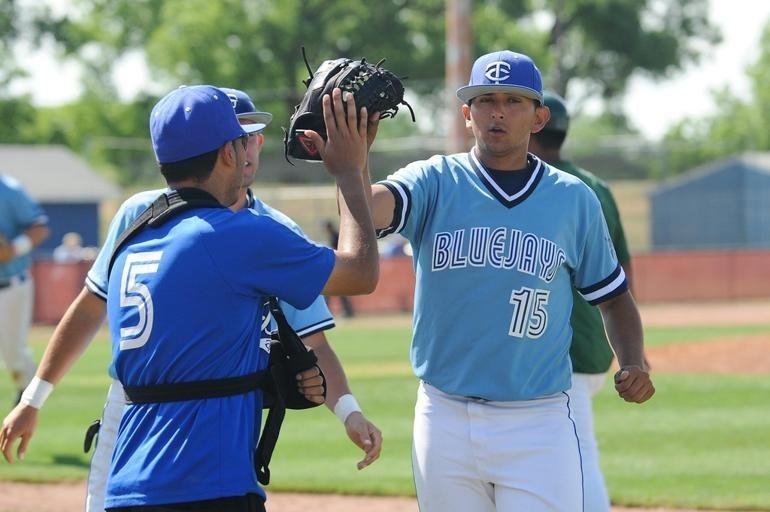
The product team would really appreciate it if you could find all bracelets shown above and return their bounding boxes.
[21,376,53,409]
[334,391,360,426]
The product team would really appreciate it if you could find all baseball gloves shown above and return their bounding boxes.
[287,59,404,159]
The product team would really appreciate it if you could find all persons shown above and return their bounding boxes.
[0,87,382,511]
[0,173,50,410]
[50,231,85,264]
[321,218,353,318]
[529,92,632,512]
[105,84,378,512]
[336,50,655,512]
